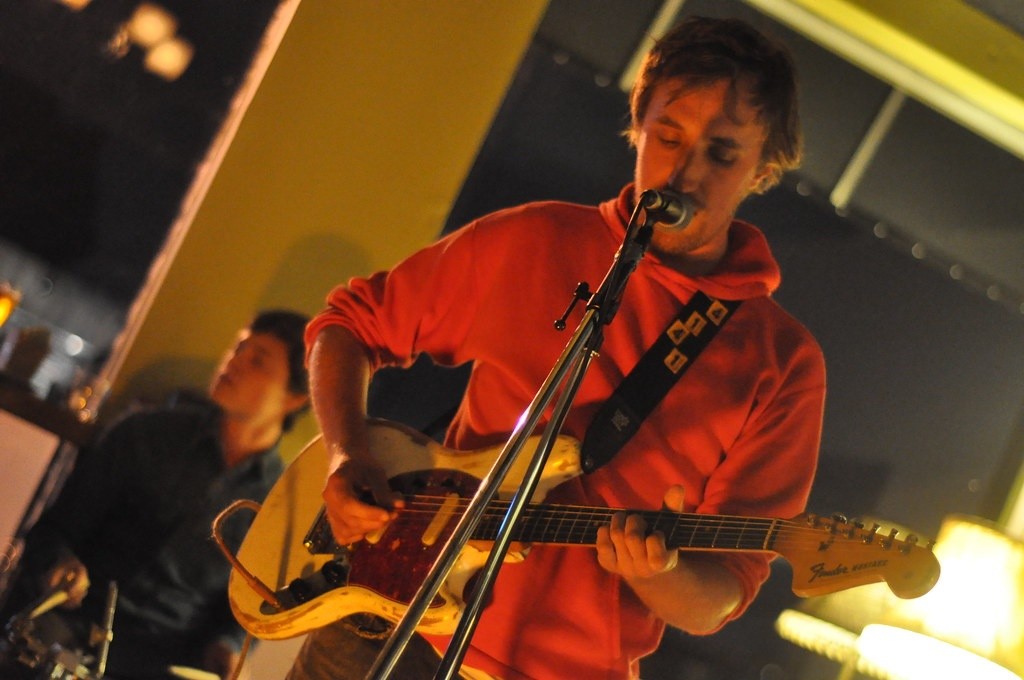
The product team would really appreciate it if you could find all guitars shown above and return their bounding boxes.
[225,417,942,643]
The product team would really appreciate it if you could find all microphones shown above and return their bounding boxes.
[639,186,693,231]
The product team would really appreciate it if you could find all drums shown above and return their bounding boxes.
[0,622,95,680]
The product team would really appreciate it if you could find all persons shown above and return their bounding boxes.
[284,16,826,680]
[0,310,312,680]
